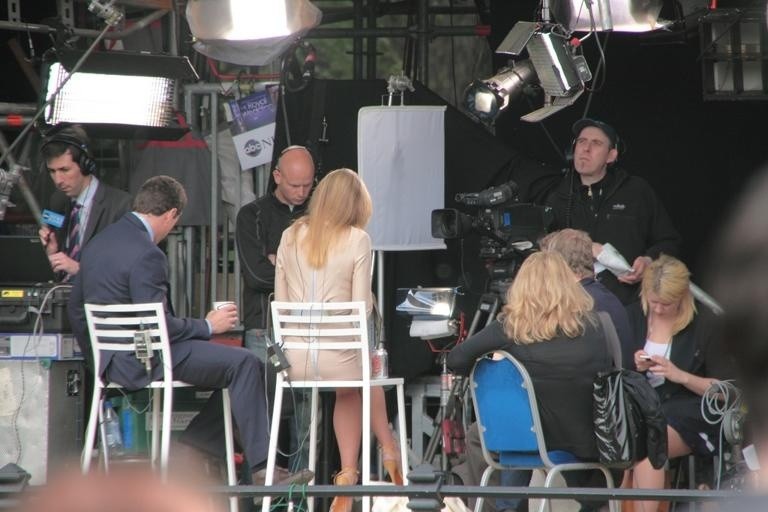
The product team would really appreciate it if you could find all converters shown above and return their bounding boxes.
[134,330,155,359]
[267,343,291,372]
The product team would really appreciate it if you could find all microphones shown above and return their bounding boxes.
[40,190,69,250]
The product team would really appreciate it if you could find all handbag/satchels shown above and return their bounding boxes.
[591,368,647,469]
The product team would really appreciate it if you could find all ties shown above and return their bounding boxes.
[68,202,81,262]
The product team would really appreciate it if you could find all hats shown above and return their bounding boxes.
[572,117,617,141]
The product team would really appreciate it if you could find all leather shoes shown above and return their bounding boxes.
[178,426,226,455]
[252,466,314,505]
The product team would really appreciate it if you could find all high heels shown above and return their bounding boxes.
[328,468,359,512]
[379,443,403,485]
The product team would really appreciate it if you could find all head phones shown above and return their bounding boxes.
[40,133,98,177]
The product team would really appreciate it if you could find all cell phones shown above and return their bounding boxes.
[640,355,650,360]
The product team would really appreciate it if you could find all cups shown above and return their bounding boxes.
[213,301,233,309]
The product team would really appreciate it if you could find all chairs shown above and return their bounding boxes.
[81,302,241,512]
[670,452,719,511]
[260,302,411,512]
[468,348,634,512]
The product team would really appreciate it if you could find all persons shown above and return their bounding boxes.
[542,228,638,372]
[236,146,325,511]
[64,174,318,505]
[447,249,626,510]
[607,253,738,511]
[544,114,683,308]
[274,168,401,510]
[681,169,767,511]
[39,126,131,285]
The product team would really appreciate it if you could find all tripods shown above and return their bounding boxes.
[422,277,514,464]
